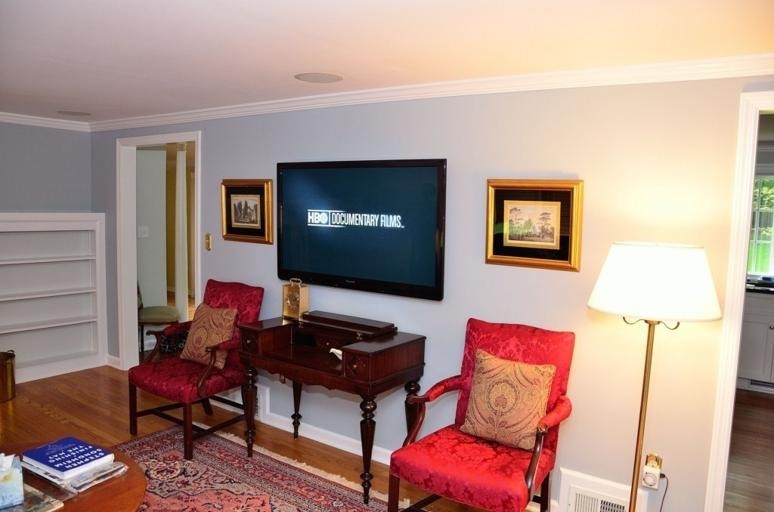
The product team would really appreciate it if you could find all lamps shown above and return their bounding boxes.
[588,241,722,512]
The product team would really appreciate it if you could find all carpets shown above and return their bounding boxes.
[108,421,429,512]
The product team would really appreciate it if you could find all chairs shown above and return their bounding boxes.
[128,279,265,460]
[388,318,575,512]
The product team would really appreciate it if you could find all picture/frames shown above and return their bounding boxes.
[220,179,274,245]
[484,178,585,272]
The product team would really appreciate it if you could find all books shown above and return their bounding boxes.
[0,481,64,512]
[22,434,127,494]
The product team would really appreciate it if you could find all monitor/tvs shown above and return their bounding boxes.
[276,158,447,302]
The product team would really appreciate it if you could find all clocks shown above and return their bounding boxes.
[281,278,310,321]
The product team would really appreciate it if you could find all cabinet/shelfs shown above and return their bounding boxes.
[736,293,774,396]
[0,213,108,385]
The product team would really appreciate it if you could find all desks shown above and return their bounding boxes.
[0,440,147,512]
[237,310,427,505]
